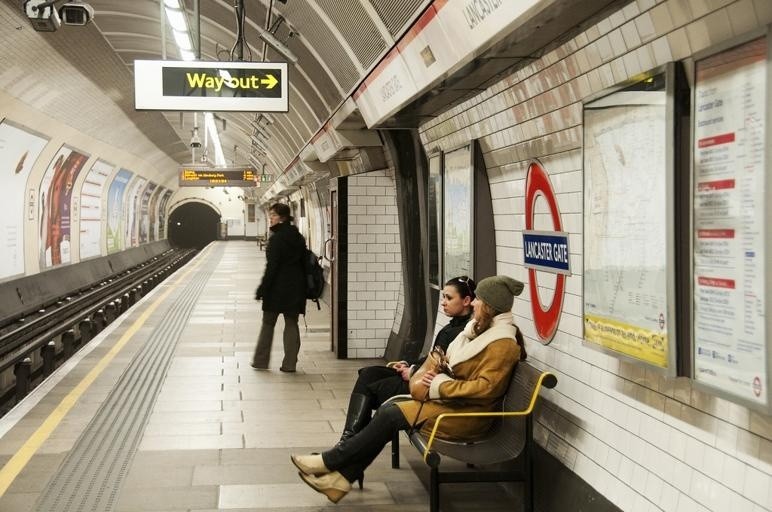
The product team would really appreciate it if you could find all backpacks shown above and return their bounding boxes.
[297,232,324,299]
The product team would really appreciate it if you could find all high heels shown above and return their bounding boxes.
[291,453,353,503]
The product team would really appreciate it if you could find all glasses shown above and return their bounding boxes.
[458,275,472,296]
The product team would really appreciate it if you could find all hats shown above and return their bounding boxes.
[474,275,524,313]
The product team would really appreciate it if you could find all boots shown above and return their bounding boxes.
[311,392,372,491]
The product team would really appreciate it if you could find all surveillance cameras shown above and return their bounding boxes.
[58,0,94,26]
[23,0,62,32]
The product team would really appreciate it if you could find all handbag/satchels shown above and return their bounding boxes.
[408,346,455,401]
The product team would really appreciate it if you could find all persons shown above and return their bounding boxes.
[247,201,305,371]
[313,276,478,490]
[288,274,524,509]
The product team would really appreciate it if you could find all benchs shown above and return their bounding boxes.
[387,361,559,512]
[255,231,268,250]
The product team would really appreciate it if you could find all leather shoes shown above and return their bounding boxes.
[249,362,296,373]
